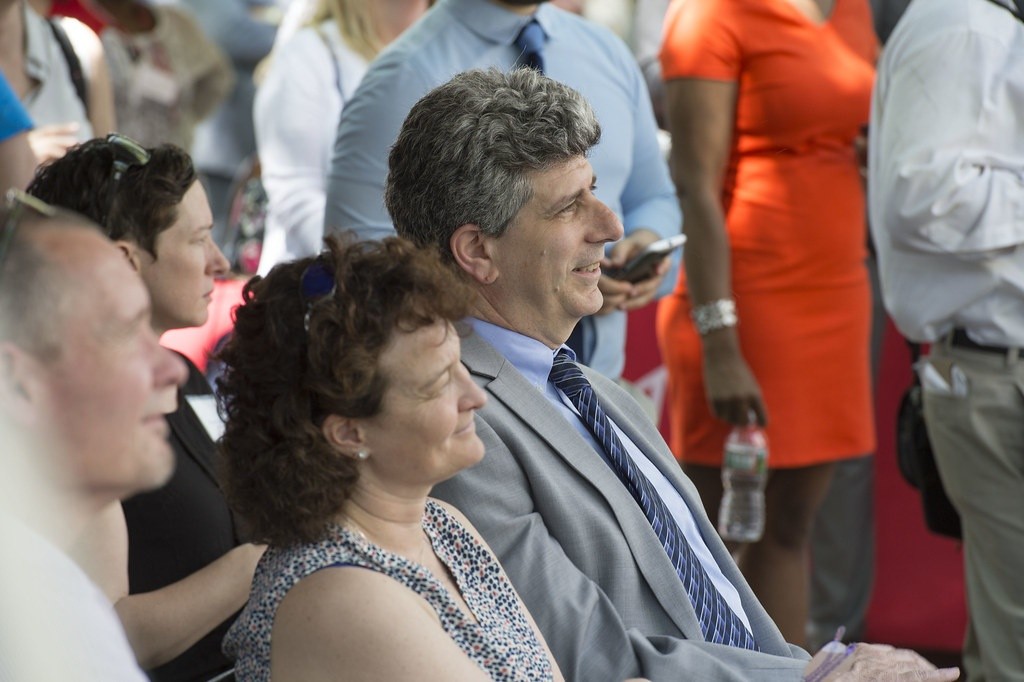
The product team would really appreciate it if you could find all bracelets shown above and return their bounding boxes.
[692,298,737,335]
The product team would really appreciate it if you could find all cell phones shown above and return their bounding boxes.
[610,233,688,283]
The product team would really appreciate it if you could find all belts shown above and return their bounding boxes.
[951,327,1024,359]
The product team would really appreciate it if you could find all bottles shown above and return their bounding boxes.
[717,412,769,542]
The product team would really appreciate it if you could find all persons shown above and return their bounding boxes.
[384,68,963,681]
[657,0,886,654]
[0,0,687,682]
[215,233,565,682]
[869,2,1024,680]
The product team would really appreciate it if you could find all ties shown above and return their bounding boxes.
[517,17,598,365]
[548,349,760,654]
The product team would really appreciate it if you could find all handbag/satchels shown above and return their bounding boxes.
[897,383,962,539]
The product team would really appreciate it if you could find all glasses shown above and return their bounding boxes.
[98,132,151,231]
